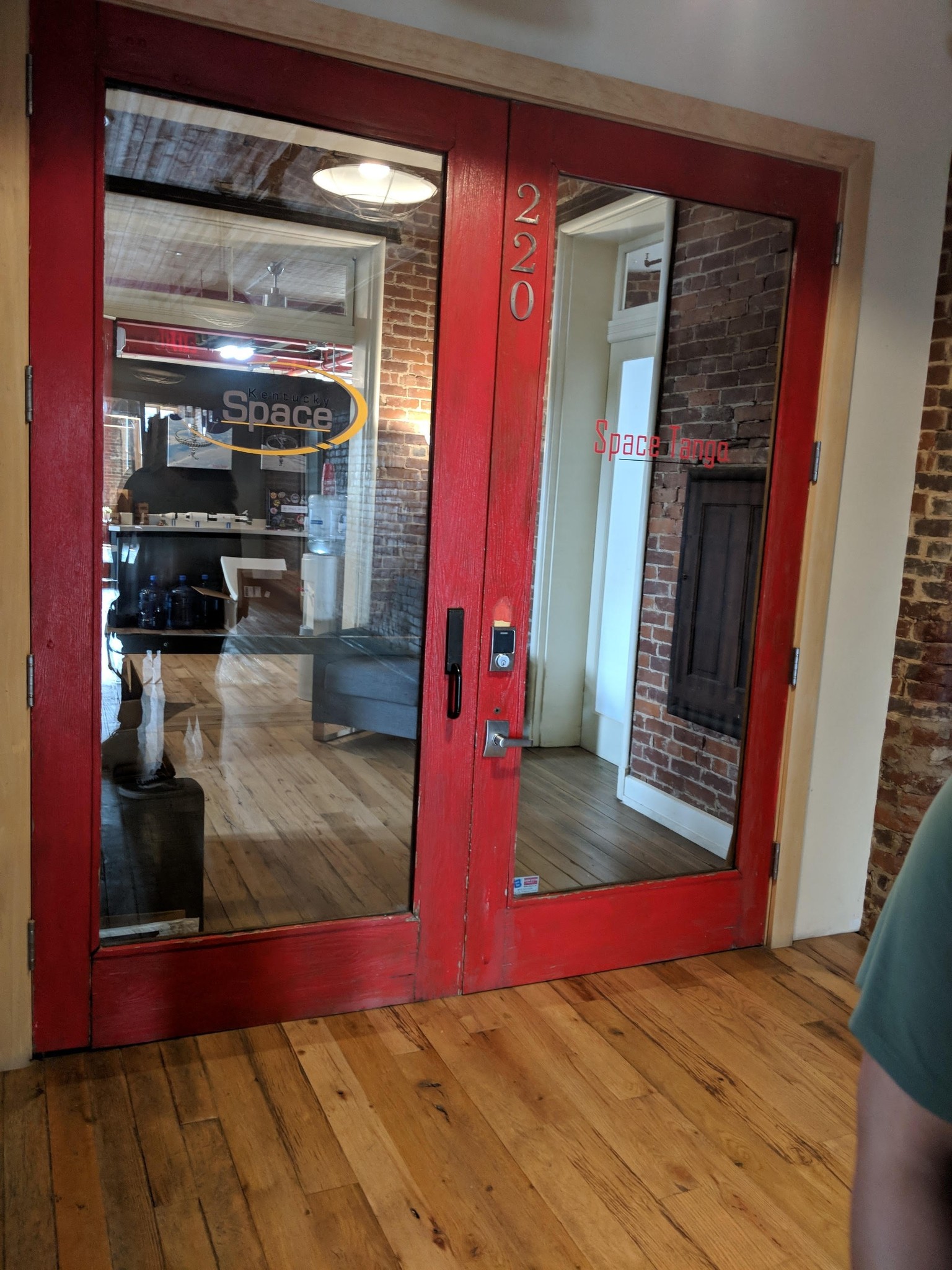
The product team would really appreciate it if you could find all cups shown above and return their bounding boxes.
[321,462,336,496]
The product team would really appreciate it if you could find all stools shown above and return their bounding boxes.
[311,626,423,745]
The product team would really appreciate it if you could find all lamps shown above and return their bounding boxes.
[262,263,287,309]
[311,151,439,224]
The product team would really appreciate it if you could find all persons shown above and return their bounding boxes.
[848,767,952,1270]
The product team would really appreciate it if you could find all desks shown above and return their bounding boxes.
[108,526,307,634]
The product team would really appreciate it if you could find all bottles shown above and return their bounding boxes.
[308,493,347,554]
[139,575,168,630]
[196,574,219,629]
[169,576,197,628]
